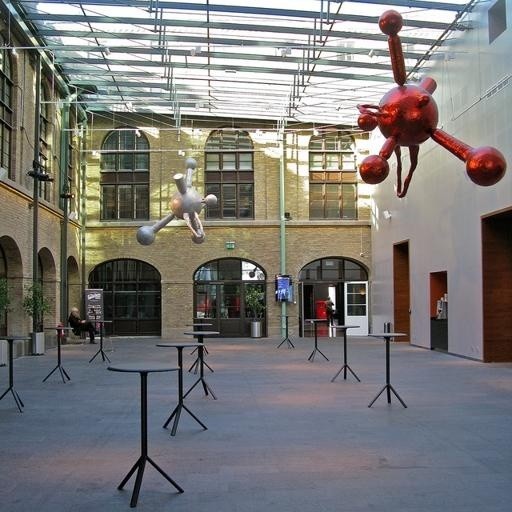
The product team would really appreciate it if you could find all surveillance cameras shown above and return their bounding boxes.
[285,212,290,218]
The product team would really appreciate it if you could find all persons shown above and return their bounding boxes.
[325,297,334,326]
[69,307,99,344]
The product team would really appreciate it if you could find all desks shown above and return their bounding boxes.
[101,316,222,510]
[275,313,409,411]
[1,319,115,414]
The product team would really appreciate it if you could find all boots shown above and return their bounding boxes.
[95,331,99,334]
[90,340,98,344]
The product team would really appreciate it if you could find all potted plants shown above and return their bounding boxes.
[0,283,53,367]
[245,285,267,338]
